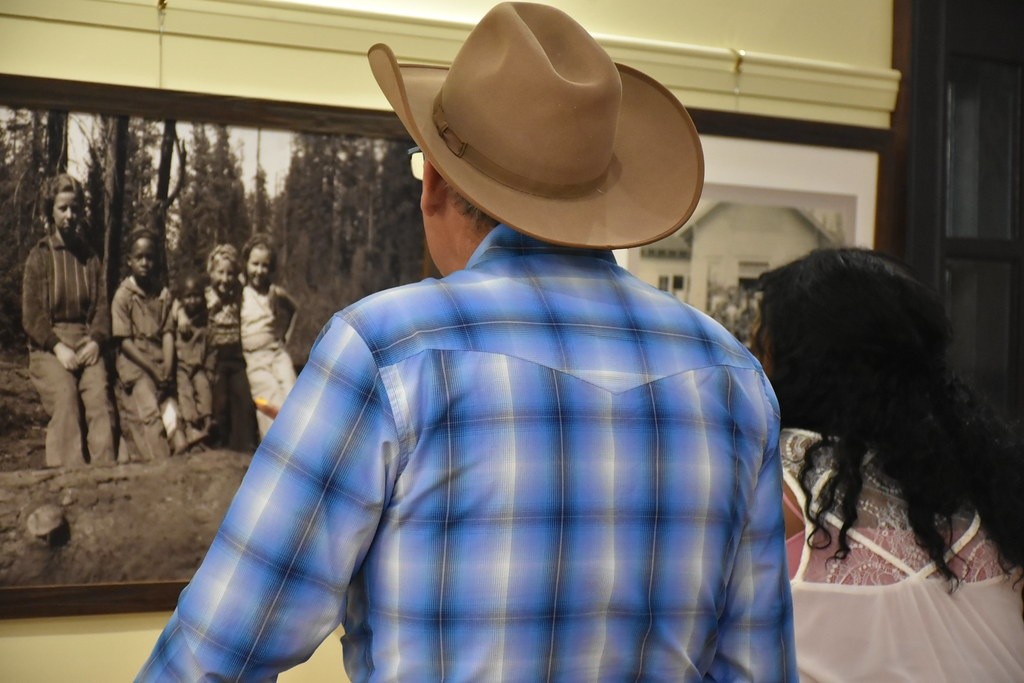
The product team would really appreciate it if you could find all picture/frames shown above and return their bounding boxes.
[618,107,899,348]
[0,72,428,613]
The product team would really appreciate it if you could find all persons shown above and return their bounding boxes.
[748,247,1024,683]
[131,1,801,683]
[22,173,298,464]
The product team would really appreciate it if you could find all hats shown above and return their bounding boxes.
[367,2,705,250]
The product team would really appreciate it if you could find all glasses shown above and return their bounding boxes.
[407,146,424,180]
